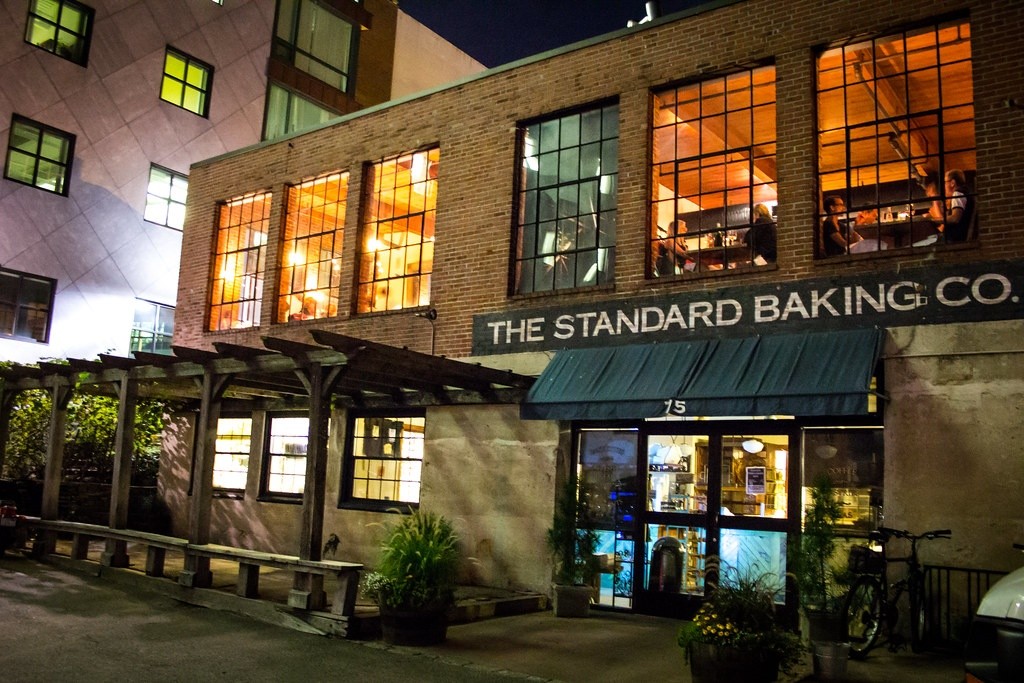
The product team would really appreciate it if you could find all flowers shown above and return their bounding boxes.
[358,505,462,618]
[677,555,808,675]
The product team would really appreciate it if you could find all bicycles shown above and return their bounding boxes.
[842,526,952,660]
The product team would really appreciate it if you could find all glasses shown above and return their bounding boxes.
[834,203,844,206]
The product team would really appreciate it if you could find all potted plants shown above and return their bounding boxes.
[784,472,865,653]
[541,473,619,617]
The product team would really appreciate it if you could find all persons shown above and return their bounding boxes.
[823,195,864,257]
[932,167,972,245]
[922,170,954,239]
[663,219,702,274]
[741,204,777,266]
[287,297,337,322]
[845,208,889,254]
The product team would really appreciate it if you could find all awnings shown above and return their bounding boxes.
[519,324,887,422]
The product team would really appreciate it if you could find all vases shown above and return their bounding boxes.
[378,605,449,646]
[687,640,780,683]
[811,640,850,683]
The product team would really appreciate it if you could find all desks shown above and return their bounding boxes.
[853,216,941,250]
[684,244,756,265]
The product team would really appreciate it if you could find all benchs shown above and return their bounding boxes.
[17,515,364,618]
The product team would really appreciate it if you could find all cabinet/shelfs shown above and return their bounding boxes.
[668,494,707,594]
[694,442,776,515]
[612,474,649,608]
